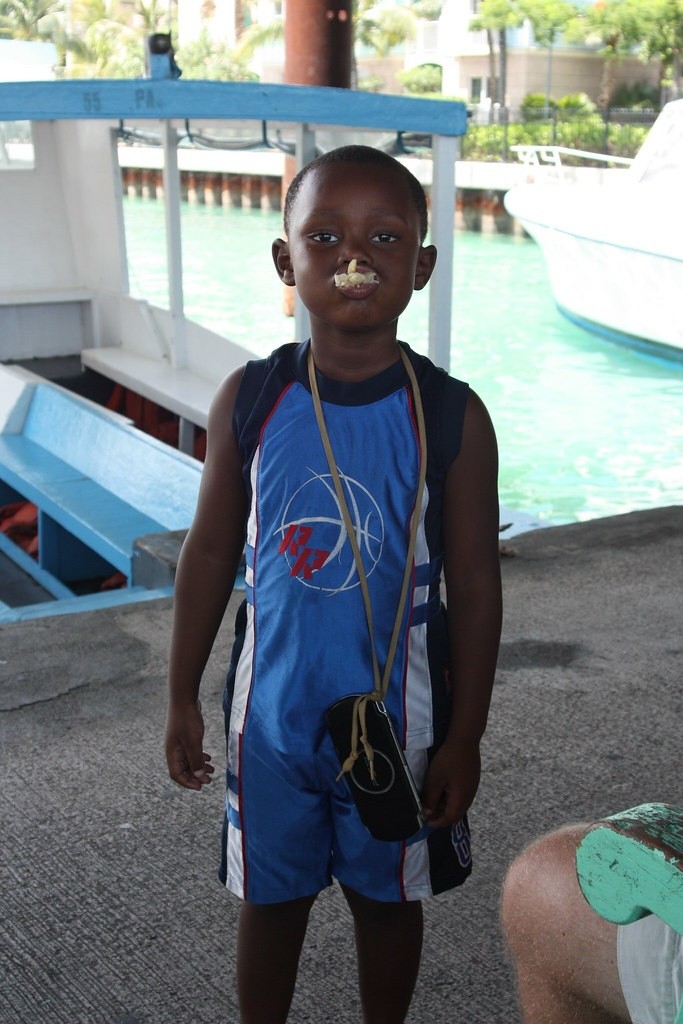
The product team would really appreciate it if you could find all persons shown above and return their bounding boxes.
[165,145,503,1024]
[501,823,683,1024]
